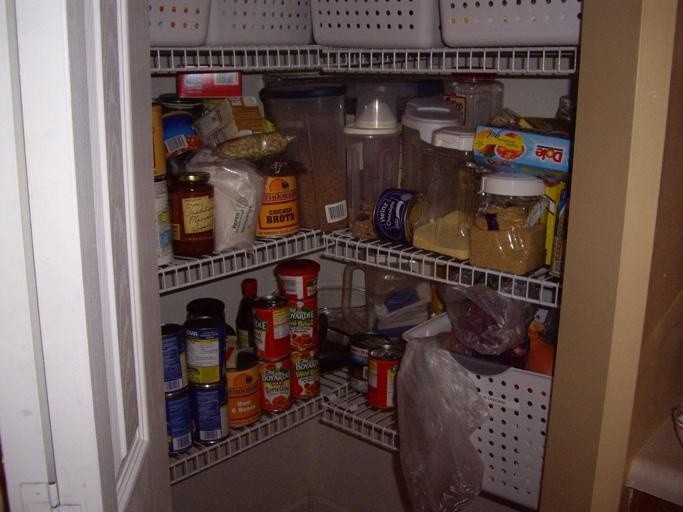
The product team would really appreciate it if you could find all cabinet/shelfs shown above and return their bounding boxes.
[322,44,579,454]
[151,45,368,483]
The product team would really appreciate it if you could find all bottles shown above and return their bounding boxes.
[183,296,233,339]
[411,126,474,261]
[260,69,356,232]
[401,95,462,181]
[342,83,400,241]
[235,277,260,345]
[466,171,547,277]
[443,71,506,129]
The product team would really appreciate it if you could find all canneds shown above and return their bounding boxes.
[151,98,169,179]
[366,346,403,411]
[162,110,202,166]
[167,171,215,256]
[153,175,174,267]
[348,331,391,394]
[253,176,300,240]
[373,188,415,244]
[160,258,320,456]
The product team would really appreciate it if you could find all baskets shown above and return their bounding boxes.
[150,1,582,47]
[150,0,582,47]
[404,312,552,509]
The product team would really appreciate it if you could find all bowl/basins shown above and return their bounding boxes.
[673,405,682,444]
[272,287,367,343]
